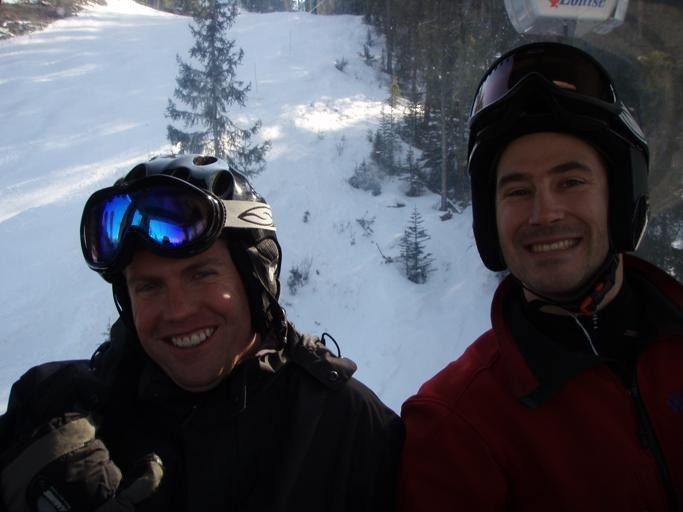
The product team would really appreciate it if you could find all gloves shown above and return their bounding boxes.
[0,411,164,511]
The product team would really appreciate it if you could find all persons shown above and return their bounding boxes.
[391,38,683,512]
[2,153,405,508]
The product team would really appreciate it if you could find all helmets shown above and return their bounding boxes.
[468,79,649,272]
[112,153,281,334]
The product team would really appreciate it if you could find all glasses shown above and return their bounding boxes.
[468,41,621,128]
[79,174,225,277]
